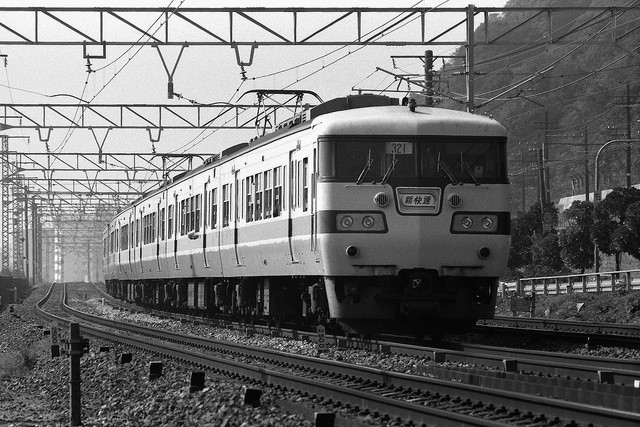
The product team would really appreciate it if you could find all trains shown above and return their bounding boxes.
[102,93,511,333]
